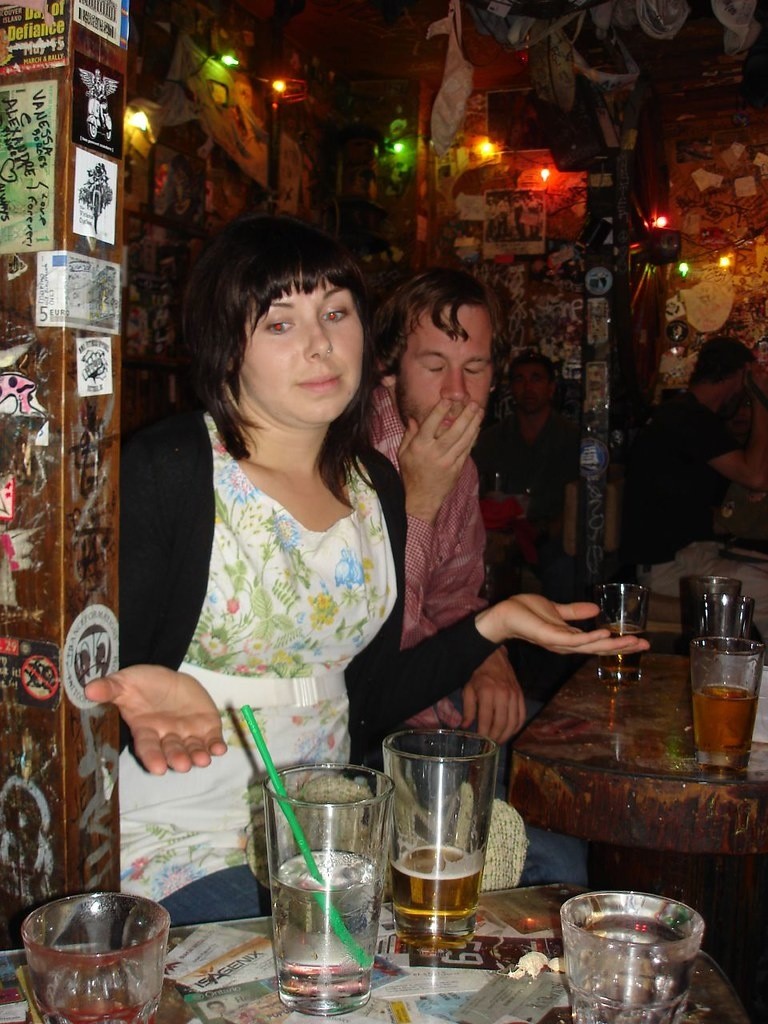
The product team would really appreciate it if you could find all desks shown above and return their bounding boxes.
[0,881,753,1024]
[509,617,767,1024]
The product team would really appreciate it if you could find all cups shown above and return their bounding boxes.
[263,764,395,1016]
[696,576,742,638]
[383,729,498,935]
[561,891,706,1024]
[597,583,650,681]
[21,892,171,1024]
[690,636,764,766]
[701,594,753,639]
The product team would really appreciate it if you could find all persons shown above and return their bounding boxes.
[365,259,622,894]
[606,335,768,666]
[475,353,595,704]
[82,214,649,931]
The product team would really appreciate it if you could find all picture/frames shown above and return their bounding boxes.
[485,87,552,153]
